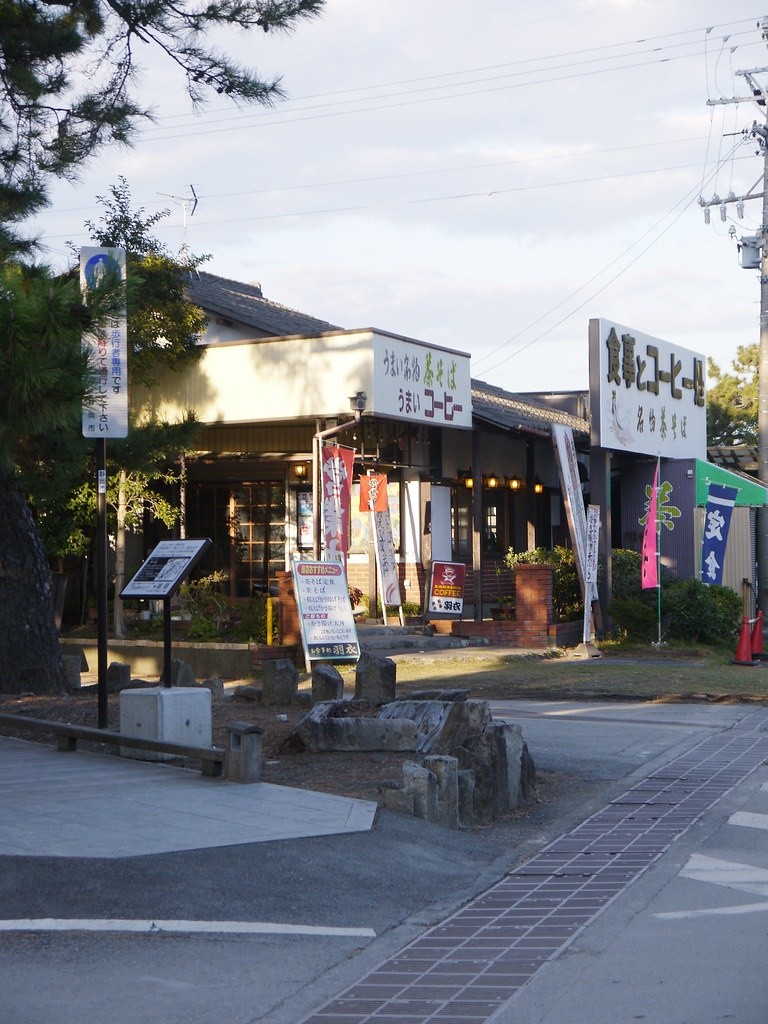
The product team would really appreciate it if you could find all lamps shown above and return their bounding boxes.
[293,465,308,480]
[486,472,500,488]
[506,474,523,492]
[533,473,545,497]
[463,466,474,489]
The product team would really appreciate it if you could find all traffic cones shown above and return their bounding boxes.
[747,610,768,660]
[730,615,762,667]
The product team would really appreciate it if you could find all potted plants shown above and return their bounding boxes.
[353,594,370,623]
[377,594,423,626]
[488,566,516,620]
